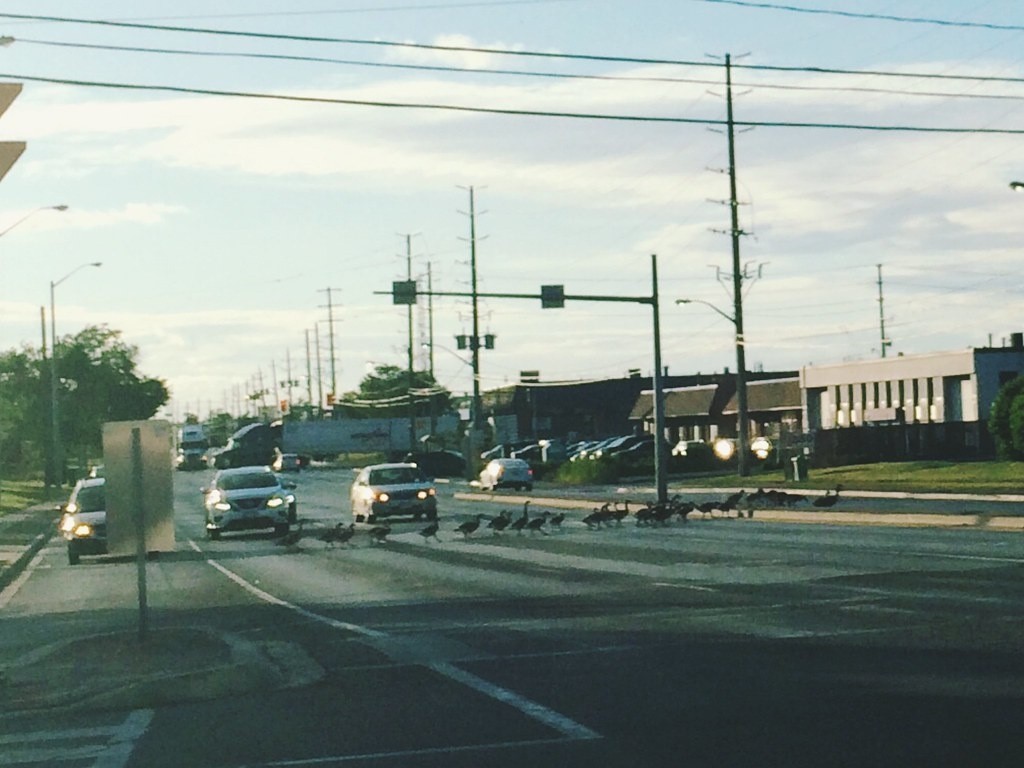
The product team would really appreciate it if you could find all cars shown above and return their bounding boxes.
[198,465,292,541]
[480,459,534,493]
[478,434,775,473]
[275,473,297,525]
[350,462,437,524]
[55,478,159,565]
[273,453,301,473]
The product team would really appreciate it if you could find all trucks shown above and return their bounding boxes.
[169,424,209,471]
[209,413,460,471]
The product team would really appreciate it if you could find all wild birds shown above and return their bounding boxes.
[368,522,391,544]
[689,485,809,519]
[336,523,356,548]
[550,513,565,528]
[419,517,443,543]
[486,510,513,532]
[275,526,305,549]
[812,486,841,507]
[582,495,695,529]
[453,514,486,538]
[508,501,530,534]
[316,522,343,549]
[524,511,551,535]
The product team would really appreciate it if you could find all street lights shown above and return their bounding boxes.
[49,260,104,489]
[418,343,481,430]
[676,297,749,477]
